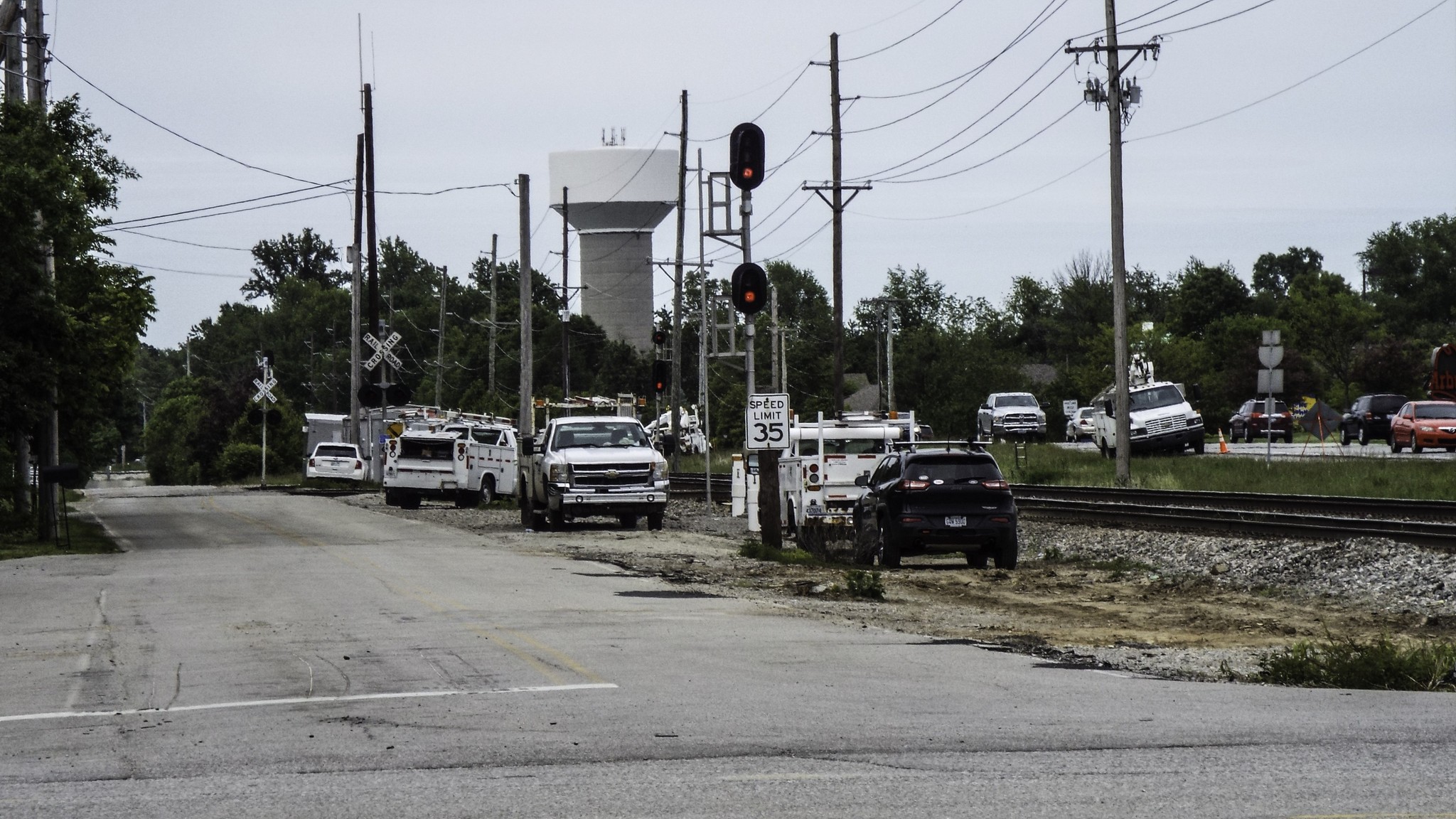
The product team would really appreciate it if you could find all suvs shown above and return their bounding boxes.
[305,442,372,488]
[1338,392,1410,446]
[1092,384,1204,459]
[853,441,1021,569]
[1228,398,1293,444]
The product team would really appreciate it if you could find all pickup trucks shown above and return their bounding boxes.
[518,415,669,533]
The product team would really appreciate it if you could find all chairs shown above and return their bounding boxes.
[611,429,628,444]
[1155,391,1174,406]
[557,430,574,448]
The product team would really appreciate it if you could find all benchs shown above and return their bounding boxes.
[572,433,634,446]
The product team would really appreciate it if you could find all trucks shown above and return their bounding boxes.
[733,413,909,555]
[382,405,528,508]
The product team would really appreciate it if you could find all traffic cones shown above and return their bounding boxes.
[1217,427,1231,455]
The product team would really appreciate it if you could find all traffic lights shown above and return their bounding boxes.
[739,131,760,182]
[656,363,665,391]
[742,270,762,310]
[657,334,663,341]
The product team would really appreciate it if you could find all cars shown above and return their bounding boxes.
[977,392,1050,444]
[918,425,935,441]
[1065,408,1099,443]
[1390,401,1456,454]
